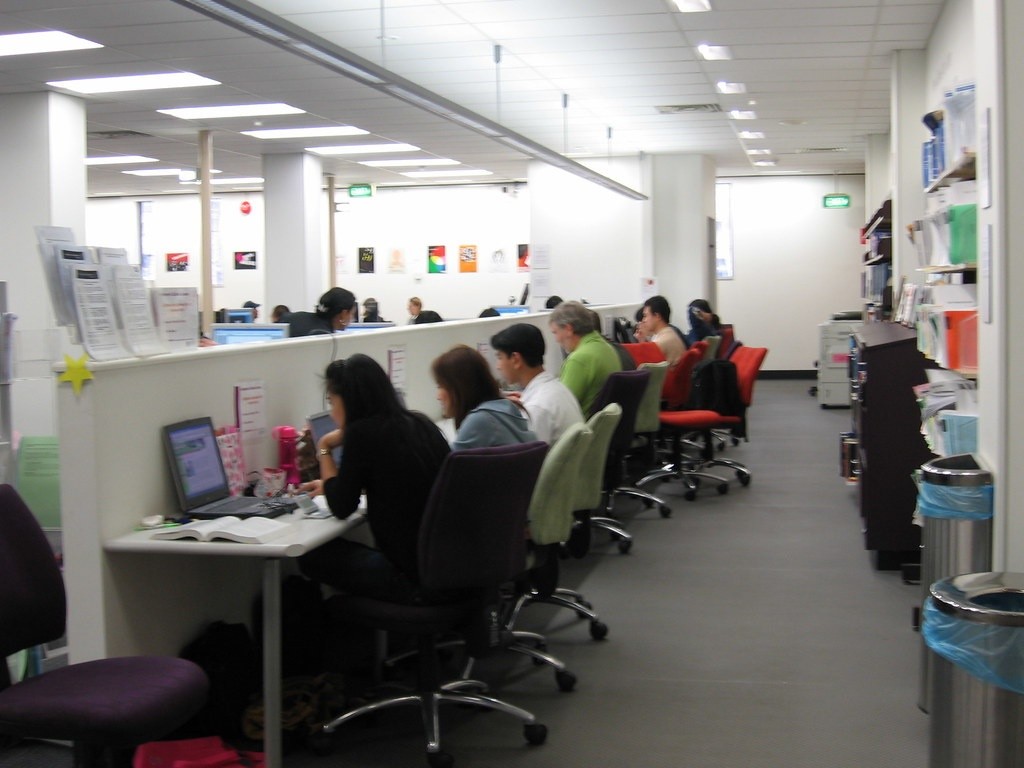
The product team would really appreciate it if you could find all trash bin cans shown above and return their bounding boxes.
[923,570,1024,768]
[918,452,992,592]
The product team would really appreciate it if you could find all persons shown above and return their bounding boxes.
[244,287,500,338]
[321,448,329,455]
[489,323,584,445]
[297,353,455,728]
[429,345,538,450]
[545,295,723,498]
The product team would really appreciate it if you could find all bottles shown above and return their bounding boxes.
[273,427,300,489]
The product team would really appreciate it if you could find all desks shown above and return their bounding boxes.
[101,419,458,768]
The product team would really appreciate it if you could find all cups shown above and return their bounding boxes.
[258,468,286,498]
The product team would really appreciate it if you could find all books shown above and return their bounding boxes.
[150,516,294,545]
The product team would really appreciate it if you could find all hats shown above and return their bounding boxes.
[244,301,261,308]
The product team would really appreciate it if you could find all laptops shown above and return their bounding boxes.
[163,416,299,519]
[307,409,343,469]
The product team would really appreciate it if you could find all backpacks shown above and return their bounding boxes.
[685,359,746,415]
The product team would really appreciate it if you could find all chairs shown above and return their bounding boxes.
[388,422,596,690]
[630,327,767,499]
[0,484,216,768]
[497,400,623,641]
[308,439,550,758]
[557,369,653,558]
[600,360,671,518]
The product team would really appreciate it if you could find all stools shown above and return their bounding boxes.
[635,411,730,503]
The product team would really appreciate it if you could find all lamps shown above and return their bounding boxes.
[171,0,649,202]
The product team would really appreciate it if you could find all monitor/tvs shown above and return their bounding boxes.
[336,322,396,333]
[209,323,291,346]
[491,305,533,316]
[221,307,254,323]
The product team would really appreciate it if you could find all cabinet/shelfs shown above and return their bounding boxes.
[848,322,940,572]
[817,320,865,408]
[863,214,892,320]
[908,152,979,380]
